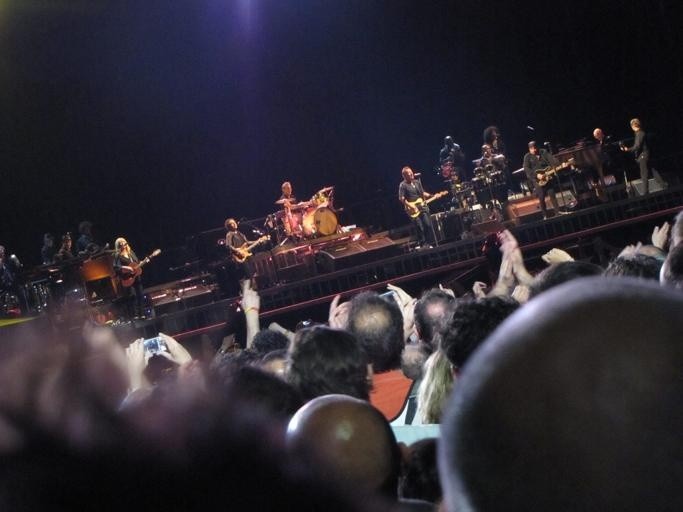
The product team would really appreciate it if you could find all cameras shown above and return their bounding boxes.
[379,290,401,305]
[142,335,169,355]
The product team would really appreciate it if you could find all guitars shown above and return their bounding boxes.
[232,235,268,263]
[404,190,448,218]
[119,248,162,288]
[533,158,575,187]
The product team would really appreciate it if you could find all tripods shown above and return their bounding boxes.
[275,201,344,246]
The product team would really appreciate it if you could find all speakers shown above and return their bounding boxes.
[178,284,212,308]
[353,236,395,262]
[122,243,127,247]
[628,178,665,197]
[316,236,366,273]
[154,287,184,317]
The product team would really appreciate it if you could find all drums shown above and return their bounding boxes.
[301,206,338,236]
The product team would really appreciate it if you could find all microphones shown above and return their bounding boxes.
[527,126,534,131]
[544,142,550,145]
[237,217,244,225]
[413,173,421,176]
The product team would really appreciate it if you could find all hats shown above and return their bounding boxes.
[44,233,55,241]
[62,231,72,240]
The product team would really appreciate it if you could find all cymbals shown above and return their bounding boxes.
[274,198,296,204]
[318,187,333,193]
